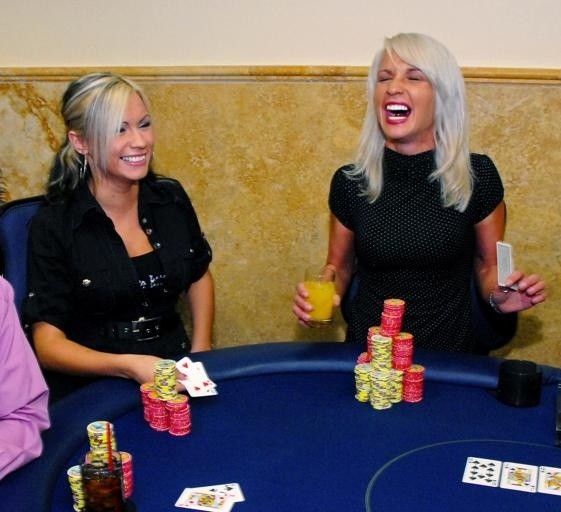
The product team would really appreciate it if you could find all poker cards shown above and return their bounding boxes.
[461,455,561,497]
[495,241,517,290]
[175,357,218,398]
[175,482,245,512]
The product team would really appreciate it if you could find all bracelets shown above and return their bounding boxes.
[489,288,504,315]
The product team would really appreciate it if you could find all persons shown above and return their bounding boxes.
[19,70,219,396]
[0,272,54,481]
[292,31,550,353]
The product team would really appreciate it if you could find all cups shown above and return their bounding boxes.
[80,449,122,512]
[303,265,335,329]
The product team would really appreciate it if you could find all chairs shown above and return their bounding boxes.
[1,197,46,330]
[339,268,361,324]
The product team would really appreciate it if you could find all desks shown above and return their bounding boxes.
[0,341,561,512]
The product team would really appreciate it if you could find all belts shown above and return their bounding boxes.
[105,313,183,344]
